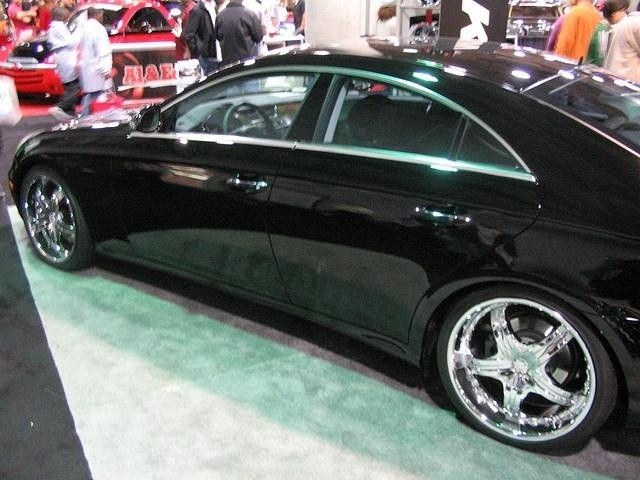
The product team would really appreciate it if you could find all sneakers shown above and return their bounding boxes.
[48,106,73,120]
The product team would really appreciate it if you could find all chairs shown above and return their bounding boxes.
[333,94,401,153]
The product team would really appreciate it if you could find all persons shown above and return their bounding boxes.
[376,5,398,35]
[544,0,640,84]
[161,0,306,95]
[0,0,118,124]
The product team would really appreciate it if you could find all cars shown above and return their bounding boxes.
[0,0,201,104]
[505,0,569,51]
[9,36,640,449]
